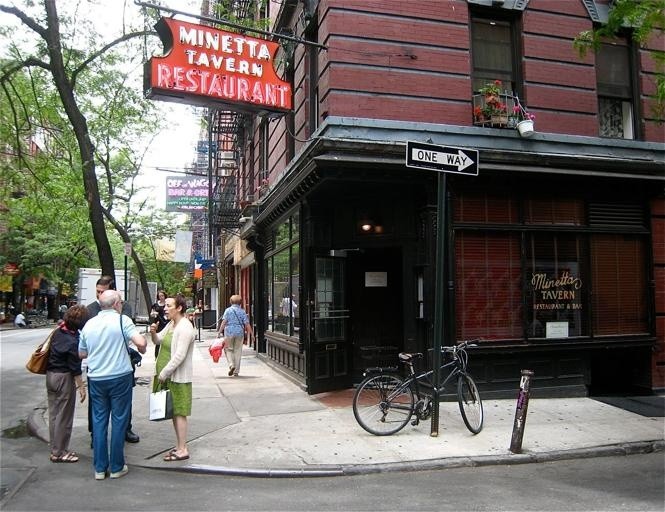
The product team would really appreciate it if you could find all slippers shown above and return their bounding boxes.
[164,454,189,461]
[167,448,177,457]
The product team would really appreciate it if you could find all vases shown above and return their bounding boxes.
[515,119,534,137]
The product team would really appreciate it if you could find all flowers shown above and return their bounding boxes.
[471,78,535,128]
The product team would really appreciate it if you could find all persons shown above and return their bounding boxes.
[151,291,171,361]
[151,295,195,461]
[58,304,67,318]
[15,312,26,328]
[216,295,253,376]
[79,275,139,449]
[46,304,88,463]
[280,294,298,317]
[79,289,147,480]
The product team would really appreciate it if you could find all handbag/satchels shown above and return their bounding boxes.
[27,325,64,375]
[129,347,143,387]
[150,383,173,421]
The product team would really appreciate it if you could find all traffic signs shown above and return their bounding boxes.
[405,139,480,177]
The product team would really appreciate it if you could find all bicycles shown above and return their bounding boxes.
[352,339,489,438]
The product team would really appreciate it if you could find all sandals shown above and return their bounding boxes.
[51,450,77,462]
[52,453,79,463]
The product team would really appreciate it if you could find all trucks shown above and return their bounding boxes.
[77,267,141,328]
[135,279,158,326]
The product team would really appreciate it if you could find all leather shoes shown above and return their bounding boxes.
[126,431,139,443]
[91,433,93,449]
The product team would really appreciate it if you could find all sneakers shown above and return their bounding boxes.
[234,373,238,377]
[110,464,129,479]
[229,368,235,376]
[95,468,109,480]
